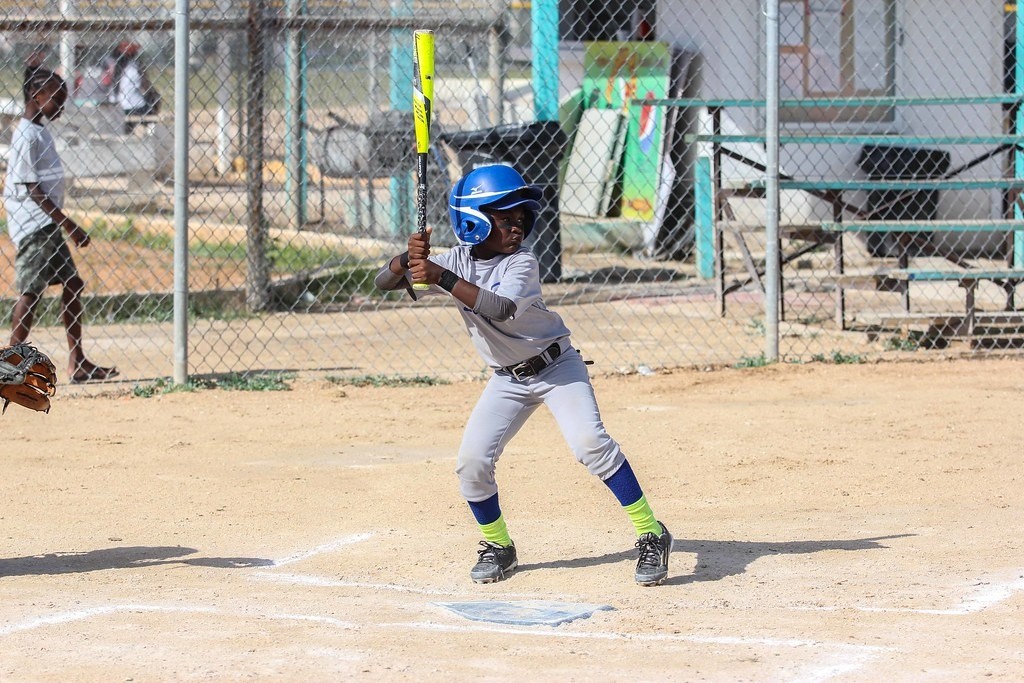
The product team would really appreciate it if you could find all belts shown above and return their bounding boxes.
[501,343,560,382]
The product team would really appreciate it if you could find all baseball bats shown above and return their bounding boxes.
[409,29,437,292]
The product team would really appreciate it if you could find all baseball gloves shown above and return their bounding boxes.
[0,344,56,413]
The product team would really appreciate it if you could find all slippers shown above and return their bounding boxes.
[70,367,119,384]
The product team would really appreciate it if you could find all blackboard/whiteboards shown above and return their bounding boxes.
[559,109,624,218]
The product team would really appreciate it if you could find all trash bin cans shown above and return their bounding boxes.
[441,120,566,285]
[867,172,941,256]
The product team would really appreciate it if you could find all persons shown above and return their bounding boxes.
[373,162,675,591]
[110,38,162,134]
[2,64,120,384]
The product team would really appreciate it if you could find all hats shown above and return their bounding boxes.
[481,196,541,211]
[119,41,140,56]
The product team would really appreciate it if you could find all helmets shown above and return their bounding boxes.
[449,166,541,247]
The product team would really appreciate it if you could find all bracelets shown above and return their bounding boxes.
[436,269,458,292]
[400,250,409,269]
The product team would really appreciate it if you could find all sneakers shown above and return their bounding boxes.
[470,540,517,585]
[634,520,675,587]
